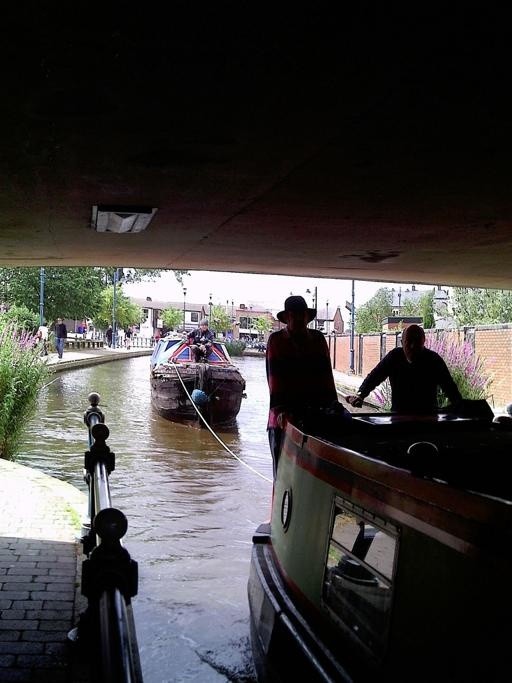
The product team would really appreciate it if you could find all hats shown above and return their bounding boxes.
[276,295,318,325]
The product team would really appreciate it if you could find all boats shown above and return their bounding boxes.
[150,338,248,428]
[247,400,512,683]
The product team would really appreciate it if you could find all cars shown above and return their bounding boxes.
[246,343,267,350]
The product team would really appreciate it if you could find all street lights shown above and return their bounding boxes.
[244,306,253,336]
[208,293,214,329]
[183,287,187,331]
[306,287,317,329]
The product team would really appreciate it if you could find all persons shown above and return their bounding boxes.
[350,322,463,447]
[34,319,50,357]
[53,317,70,359]
[264,294,344,523]
[103,323,115,348]
[153,330,160,345]
[123,328,132,350]
[184,317,216,364]
